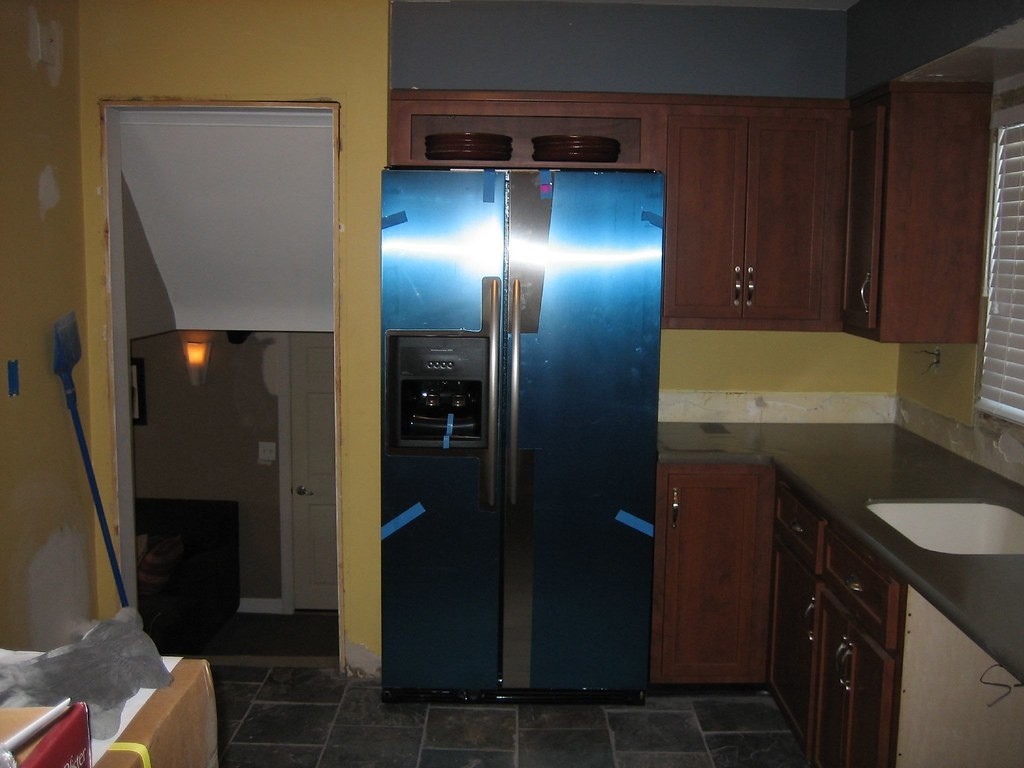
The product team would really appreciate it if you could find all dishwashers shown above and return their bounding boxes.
[896,583,1024,768]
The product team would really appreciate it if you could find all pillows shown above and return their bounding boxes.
[134,532,184,595]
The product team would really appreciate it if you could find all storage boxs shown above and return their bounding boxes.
[0,648,222,768]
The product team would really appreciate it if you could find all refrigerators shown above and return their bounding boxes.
[382,163,667,709]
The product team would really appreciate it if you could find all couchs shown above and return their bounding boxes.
[133,494,240,657]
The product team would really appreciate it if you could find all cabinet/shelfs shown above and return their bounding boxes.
[387,82,992,345]
[655,465,1024,768]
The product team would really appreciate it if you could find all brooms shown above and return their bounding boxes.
[52,310,131,608]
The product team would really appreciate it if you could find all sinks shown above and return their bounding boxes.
[864,496,1024,555]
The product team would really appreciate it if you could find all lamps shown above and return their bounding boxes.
[183,342,210,386]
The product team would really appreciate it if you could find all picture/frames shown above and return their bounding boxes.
[130,356,147,427]
